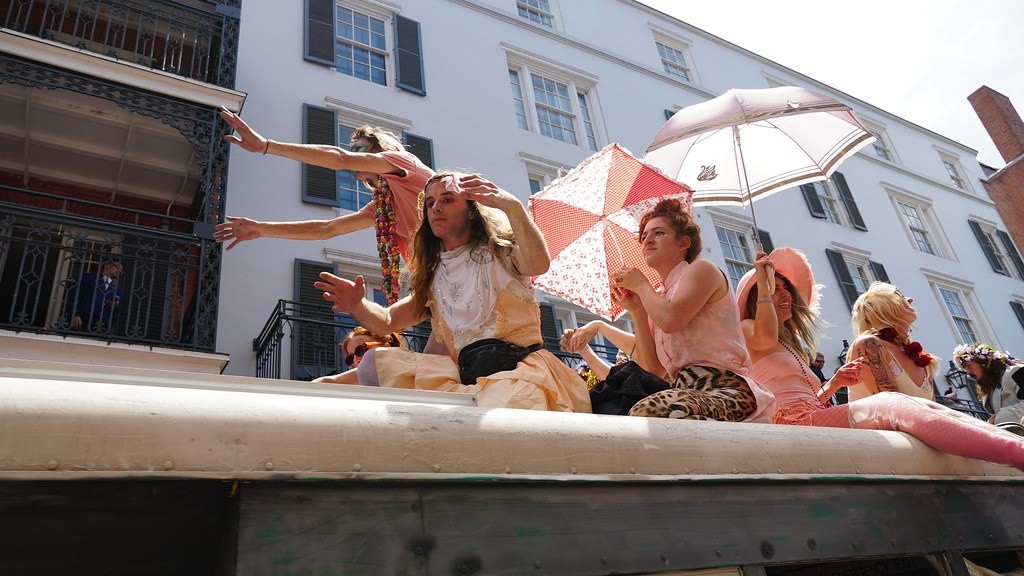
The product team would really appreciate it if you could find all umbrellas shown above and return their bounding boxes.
[645,87,880,297]
[528,142,695,322]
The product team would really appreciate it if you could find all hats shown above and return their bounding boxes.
[736,248,814,336]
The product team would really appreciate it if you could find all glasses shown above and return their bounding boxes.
[815,359,827,364]
[344,342,385,364]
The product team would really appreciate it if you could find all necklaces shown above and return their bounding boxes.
[778,339,833,407]
[373,178,403,306]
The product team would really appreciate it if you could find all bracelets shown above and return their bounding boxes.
[756,298,774,306]
[262,138,269,156]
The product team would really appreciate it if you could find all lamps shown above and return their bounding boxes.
[837,338,854,366]
[944,360,971,391]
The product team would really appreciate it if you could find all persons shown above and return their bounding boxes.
[310,321,408,383]
[807,352,834,405]
[609,199,777,424]
[847,281,939,402]
[215,104,437,263]
[950,343,1024,437]
[562,326,638,380]
[736,246,1024,467]
[314,172,550,384]
[72,260,124,335]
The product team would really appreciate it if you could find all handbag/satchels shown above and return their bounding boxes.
[457,337,528,387]
